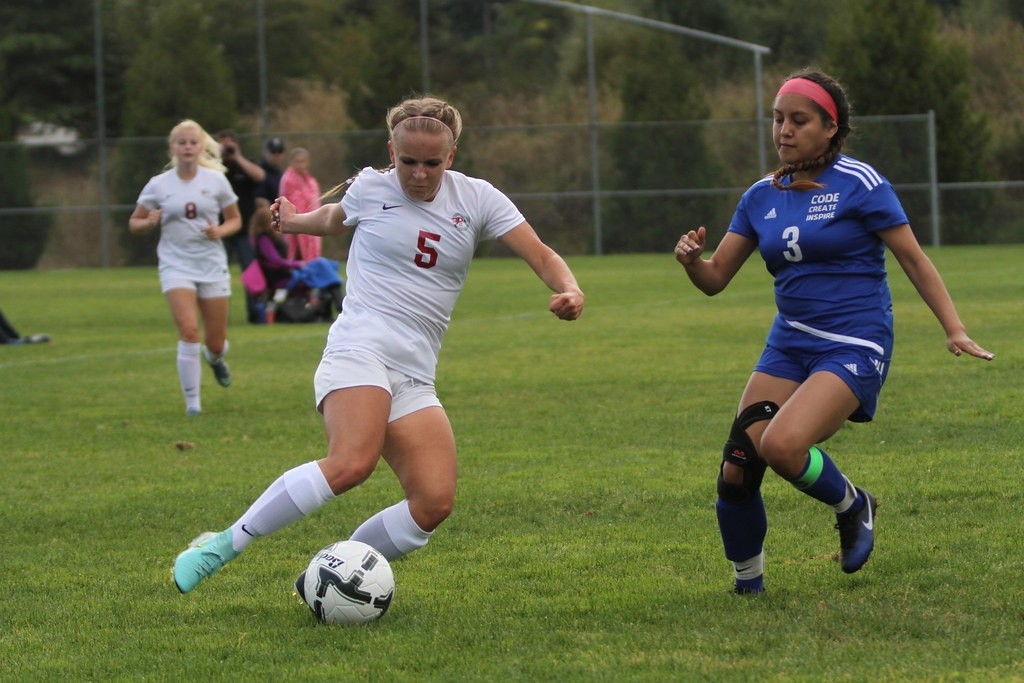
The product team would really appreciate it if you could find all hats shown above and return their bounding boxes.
[269,138,284,152]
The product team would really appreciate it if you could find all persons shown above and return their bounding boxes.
[218,129,325,322]
[128,119,243,416]
[173,94,589,610]
[674,68,997,596]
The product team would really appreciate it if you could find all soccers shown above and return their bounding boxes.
[304,539,394,625]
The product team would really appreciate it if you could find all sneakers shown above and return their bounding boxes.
[203,348,231,387]
[172,530,230,594]
[835,486,879,575]
